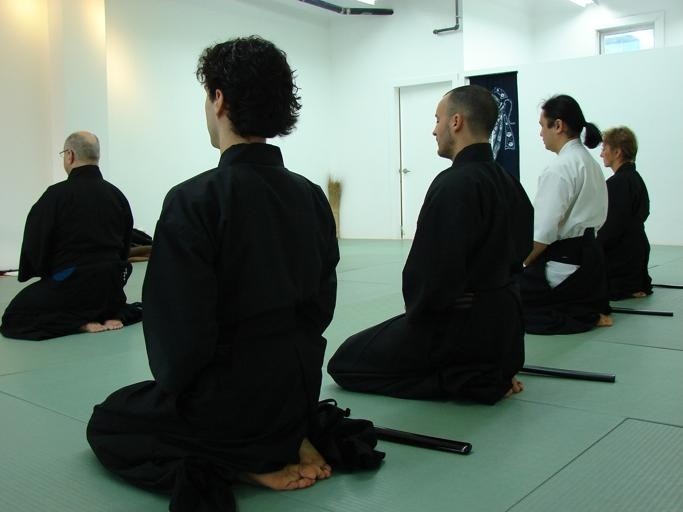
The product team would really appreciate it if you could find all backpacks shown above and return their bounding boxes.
[131,228,155,246]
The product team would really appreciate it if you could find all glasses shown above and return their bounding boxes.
[58,149,69,158]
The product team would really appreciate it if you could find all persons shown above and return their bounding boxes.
[599,125,653,301]
[87,34,341,491]
[327,85,534,403]
[0,131,134,341]
[523,95,613,335]
[168,467,236,512]
